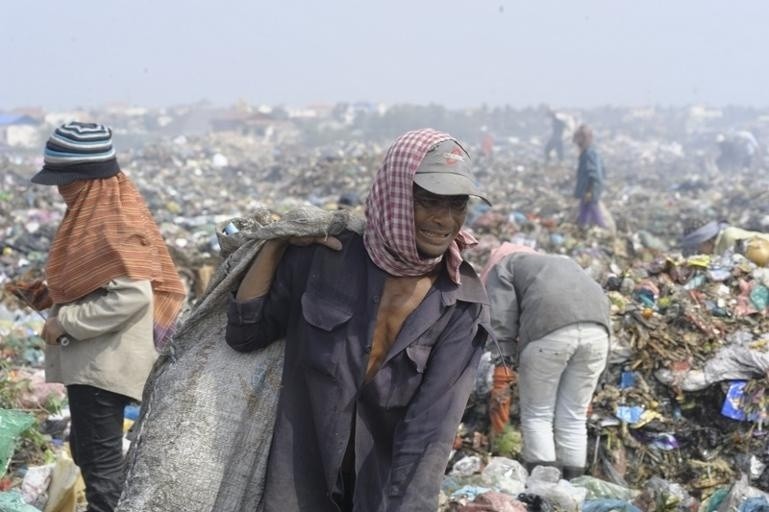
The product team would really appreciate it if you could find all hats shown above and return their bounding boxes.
[31,120,121,186]
[413,138,493,207]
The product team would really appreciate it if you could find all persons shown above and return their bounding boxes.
[10,122,186,512]
[481,241,612,482]
[540,104,618,230]
[225,126,492,512]
